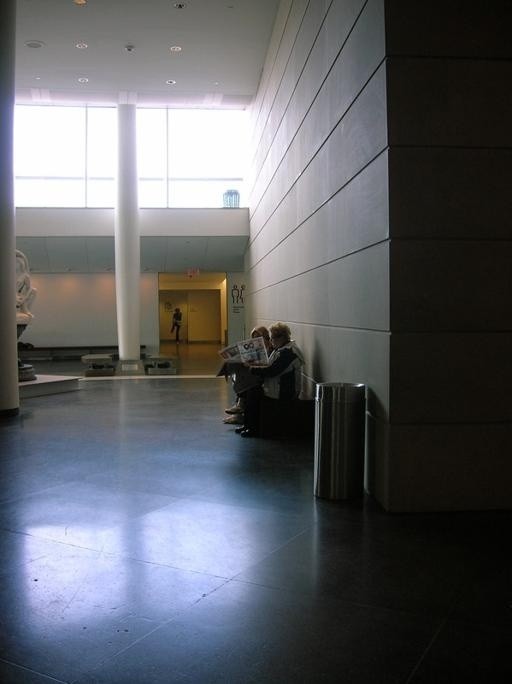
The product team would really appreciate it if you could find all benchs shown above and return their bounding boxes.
[145,353,179,375]
[273,389,314,439]
[80,351,115,377]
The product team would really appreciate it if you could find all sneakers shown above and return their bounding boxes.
[223,404,248,436]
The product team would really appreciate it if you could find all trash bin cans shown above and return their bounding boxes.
[313,382,365,502]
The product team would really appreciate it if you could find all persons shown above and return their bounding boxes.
[222,326,273,423]
[170,307,183,344]
[235,320,305,437]
[16,255,33,317]
